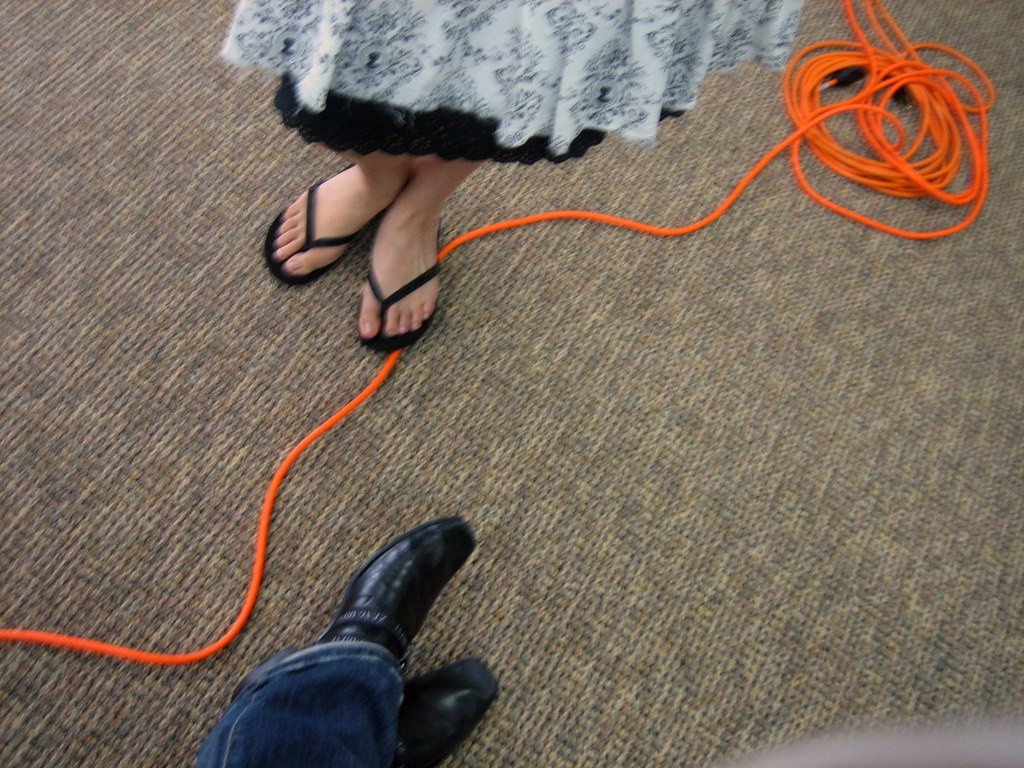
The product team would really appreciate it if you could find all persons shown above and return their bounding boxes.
[217,0,806,351]
[193,512,499,768]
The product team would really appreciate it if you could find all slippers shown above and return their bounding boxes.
[265,163,411,287]
[358,215,442,352]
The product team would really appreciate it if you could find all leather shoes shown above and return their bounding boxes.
[313,516,476,661]
[389,660,499,768]
